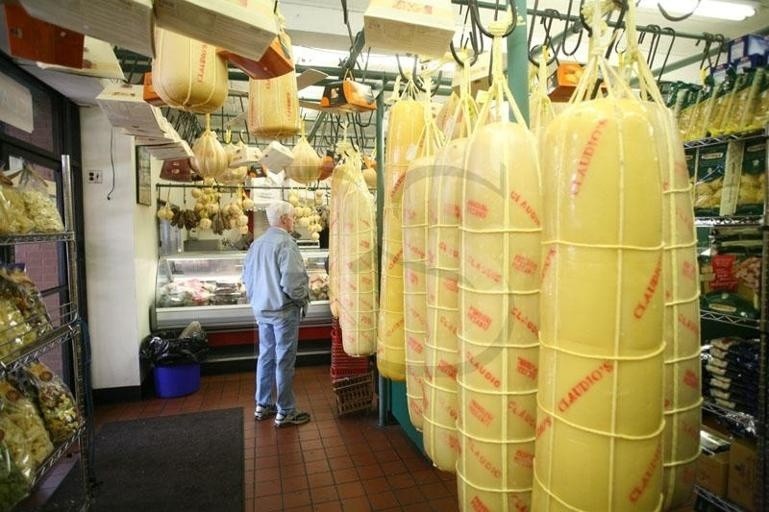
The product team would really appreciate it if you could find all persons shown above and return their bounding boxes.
[241,199,313,430]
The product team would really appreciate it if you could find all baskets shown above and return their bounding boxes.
[330,319,372,415]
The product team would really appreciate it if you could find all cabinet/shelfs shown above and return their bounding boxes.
[1,142,104,512]
[682,125,769,510]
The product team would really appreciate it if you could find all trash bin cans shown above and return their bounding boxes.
[139,327,209,398]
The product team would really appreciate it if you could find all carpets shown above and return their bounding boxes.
[38,405,247,510]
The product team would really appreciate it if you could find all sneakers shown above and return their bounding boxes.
[275,408,310,428]
[254,403,278,420]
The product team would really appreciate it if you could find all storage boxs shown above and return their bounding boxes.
[728,437,760,510]
[694,418,731,499]
